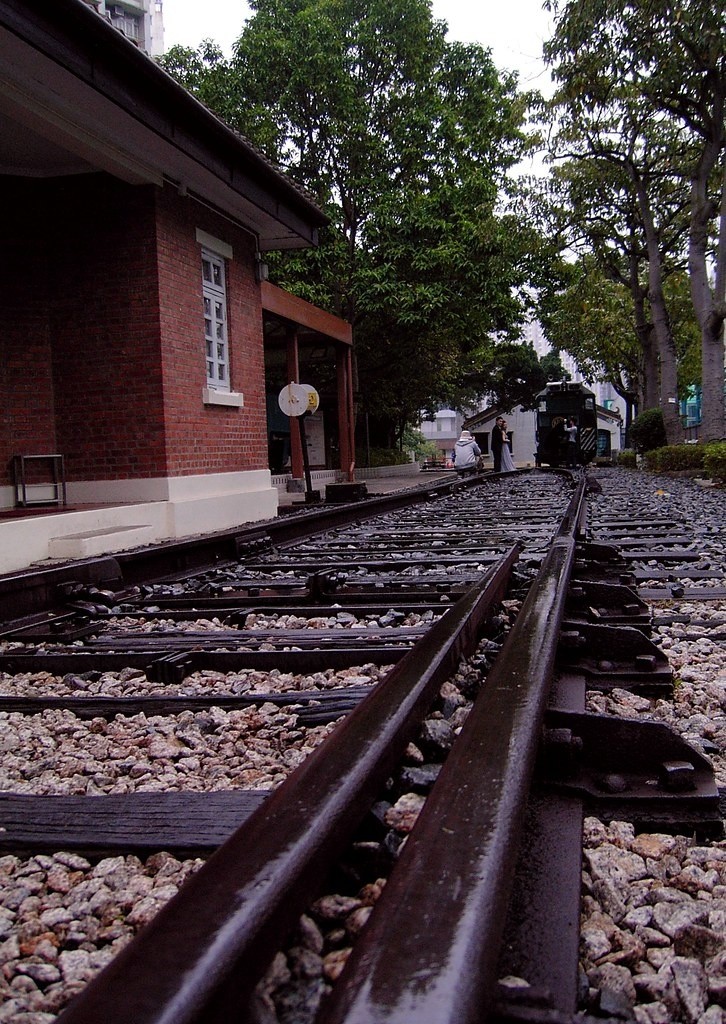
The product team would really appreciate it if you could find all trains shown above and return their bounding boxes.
[533,377,599,468]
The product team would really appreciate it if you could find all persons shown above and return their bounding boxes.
[490,416,503,472]
[563,416,578,468]
[453,431,483,478]
[501,420,515,472]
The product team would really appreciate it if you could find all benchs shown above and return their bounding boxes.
[422,465,446,467]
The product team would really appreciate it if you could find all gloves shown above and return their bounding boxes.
[564,418,567,424]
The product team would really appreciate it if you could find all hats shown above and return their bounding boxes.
[460,431,472,439]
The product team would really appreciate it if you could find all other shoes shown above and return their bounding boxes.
[458,475,462,479]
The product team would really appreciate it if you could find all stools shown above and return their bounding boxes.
[12,453,67,507]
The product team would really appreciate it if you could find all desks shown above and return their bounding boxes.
[422,461,446,469]
